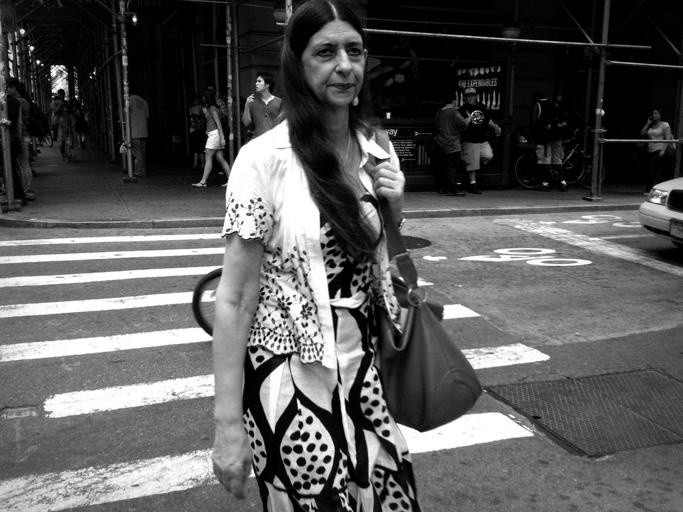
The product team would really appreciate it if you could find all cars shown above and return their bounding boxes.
[639,177,683,247]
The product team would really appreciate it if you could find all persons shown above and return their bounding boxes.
[640,105,677,192]
[211,0,421,512]
[0,70,287,206]
[529,90,568,191]
[434,93,474,195]
[457,87,501,193]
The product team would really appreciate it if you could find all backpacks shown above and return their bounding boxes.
[16,96,49,137]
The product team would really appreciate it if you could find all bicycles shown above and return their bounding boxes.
[40,127,53,149]
[511,123,605,192]
[191,263,224,337]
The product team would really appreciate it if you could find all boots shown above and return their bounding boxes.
[470,183,483,195]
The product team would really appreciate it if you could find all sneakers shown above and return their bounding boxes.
[538,184,551,192]
[221,183,228,187]
[438,188,448,194]
[446,191,465,197]
[560,183,568,192]
[191,182,208,187]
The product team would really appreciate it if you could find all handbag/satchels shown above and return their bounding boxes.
[374,286,482,433]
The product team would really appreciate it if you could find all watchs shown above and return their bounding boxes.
[397,215,405,230]
